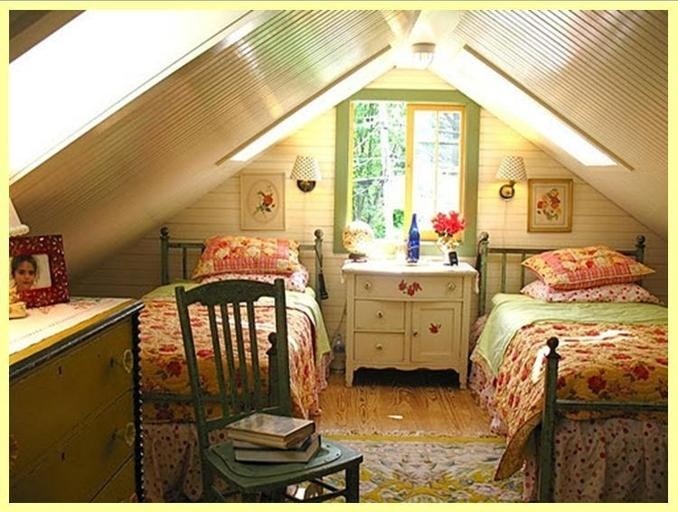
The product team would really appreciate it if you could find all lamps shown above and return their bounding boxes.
[495,156,527,199]
[290,155,321,192]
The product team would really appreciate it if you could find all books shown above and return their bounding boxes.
[226,413,322,463]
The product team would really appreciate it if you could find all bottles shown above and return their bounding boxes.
[407,213,420,261]
[332,334,345,373]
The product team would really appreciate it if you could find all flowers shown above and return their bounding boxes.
[431,211,464,238]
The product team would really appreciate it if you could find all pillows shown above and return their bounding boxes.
[520,246,656,290]
[201,263,309,293]
[191,234,300,279]
[520,279,664,306]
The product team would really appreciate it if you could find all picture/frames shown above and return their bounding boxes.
[527,178,574,233]
[10,235,70,310]
[240,171,286,232]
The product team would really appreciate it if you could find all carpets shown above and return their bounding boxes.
[142,421,523,503]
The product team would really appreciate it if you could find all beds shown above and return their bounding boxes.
[139,227,335,502]
[469,232,668,503]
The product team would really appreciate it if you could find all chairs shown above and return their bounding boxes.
[175,278,363,503]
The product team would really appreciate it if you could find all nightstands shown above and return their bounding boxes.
[341,258,480,390]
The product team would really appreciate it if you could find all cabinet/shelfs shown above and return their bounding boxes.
[10,296,147,502]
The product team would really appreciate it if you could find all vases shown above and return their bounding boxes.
[437,236,458,256]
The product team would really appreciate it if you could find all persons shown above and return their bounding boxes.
[11,255,38,293]
